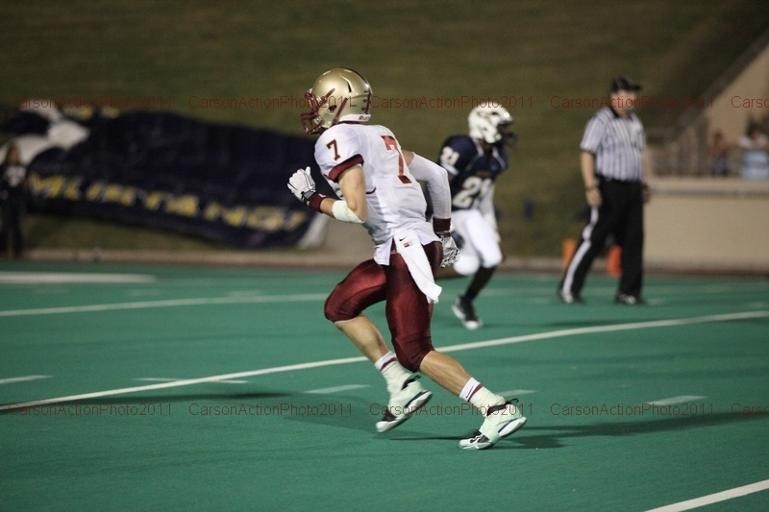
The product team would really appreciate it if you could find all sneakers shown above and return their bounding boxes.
[556,289,586,304]
[615,289,647,305]
[456,400,528,451]
[450,295,482,332]
[374,374,432,432]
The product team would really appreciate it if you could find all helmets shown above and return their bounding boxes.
[468,101,515,145]
[295,66,375,131]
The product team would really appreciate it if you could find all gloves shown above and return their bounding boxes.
[285,165,315,205]
[438,231,460,270]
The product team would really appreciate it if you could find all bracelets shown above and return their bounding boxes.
[307,191,326,212]
[431,216,451,231]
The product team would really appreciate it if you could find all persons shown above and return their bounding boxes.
[0,145,30,261]
[435,101,515,332]
[286,67,525,452]
[562,188,652,278]
[557,76,654,306]
[704,119,769,181]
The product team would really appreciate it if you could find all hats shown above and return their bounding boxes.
[609,75,642,92]
